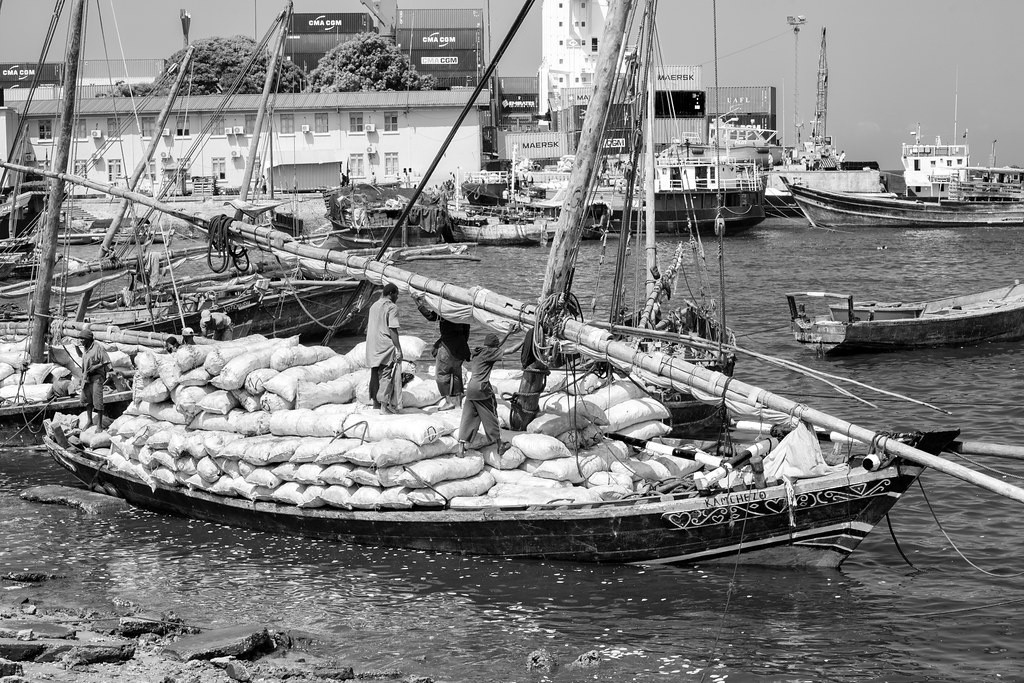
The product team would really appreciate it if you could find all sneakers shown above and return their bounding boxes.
[454,450,464,459]
[496,441,510,454]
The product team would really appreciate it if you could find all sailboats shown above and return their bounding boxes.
[1,1,1024,569]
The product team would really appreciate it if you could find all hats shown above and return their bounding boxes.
[200,310,210,322]
[77,328,93,340]
[181,327,198,335]
[483,332,499,346]
[54,366,70,378]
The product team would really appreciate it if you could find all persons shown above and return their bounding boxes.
[429,171,463,209]
[181,327,198,345]
[840,151,846,169]
[785,156,790,166]
[410,292,472,411]
[370,172,377,185]
[453,333,526,459]
[199,309,235,341]
[799,156,808,170]
[518,169,529,189]
[165,337,181,353]
[75,329,113,433]
[366,284,403,415]
[341,173,348,186]
[407,168,413,186]
[781,150,787,163]
[261,175,269,194]
[767,153,773,167]
[789,156,792,164]
[824,149,830,156]
[808,151,816,172]
[107,248,116,261]
[402,168,410,185]
[524,170,534,183]
[835,154,841,170]
